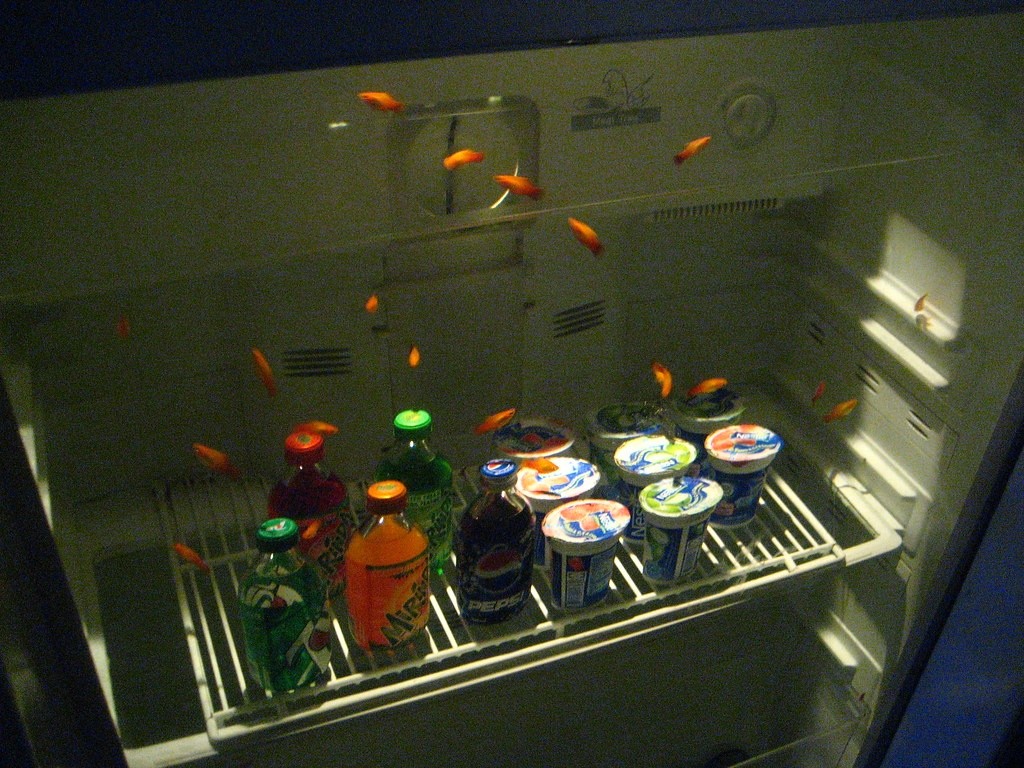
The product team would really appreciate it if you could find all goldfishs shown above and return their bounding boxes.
[115,88,930,575]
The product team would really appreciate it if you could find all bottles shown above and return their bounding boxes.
[344,479,431,655]
[453,458,537,623]
[370,409,454,578]
[239,517,335,691]
[269,430,361,601]
